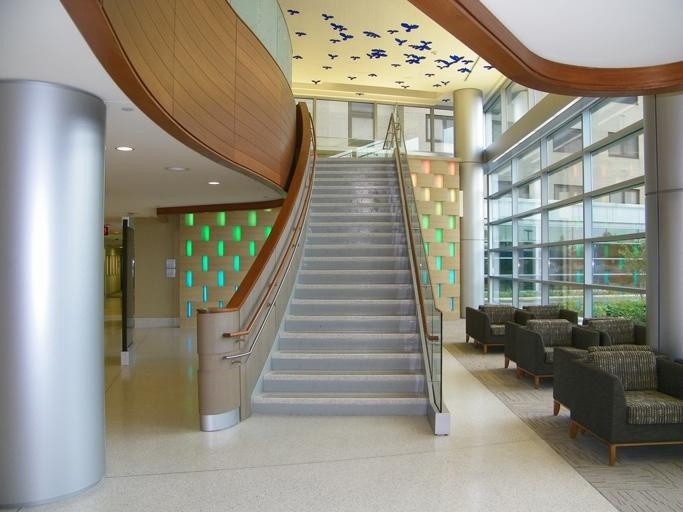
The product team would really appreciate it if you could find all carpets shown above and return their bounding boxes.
[443,342,682,512]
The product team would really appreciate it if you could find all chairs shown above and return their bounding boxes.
[465,303,682,465]
